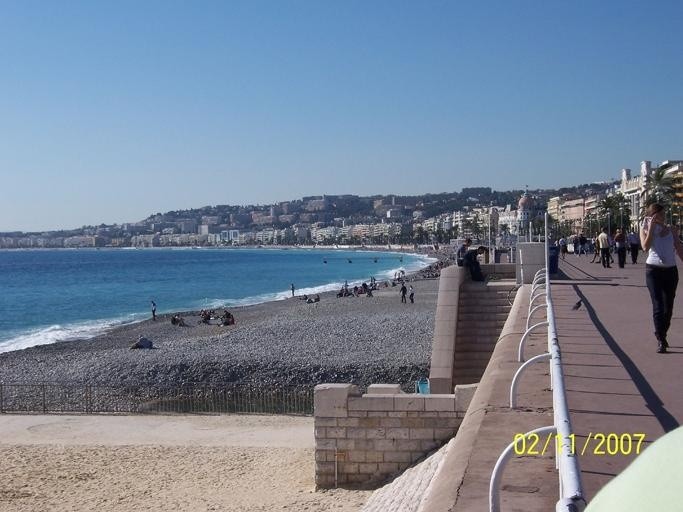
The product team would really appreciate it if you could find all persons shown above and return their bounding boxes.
[300,294,320,303]
[457,238,472,266]
[638,203,683,353]
[290,283,295,297]
[170,313,185,326]
[573,227,640,269]
[151,300,157,320]
[553,235,567,260]
[336,270,414,303]
[667,222,681,256]
[199,308,231,327]
[462,245,490,284]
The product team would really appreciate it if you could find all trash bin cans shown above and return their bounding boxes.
[506,246,516,263]
[550,246,559,273]
[418,380,428,394]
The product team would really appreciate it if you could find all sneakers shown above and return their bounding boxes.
[657,338,668,352]
